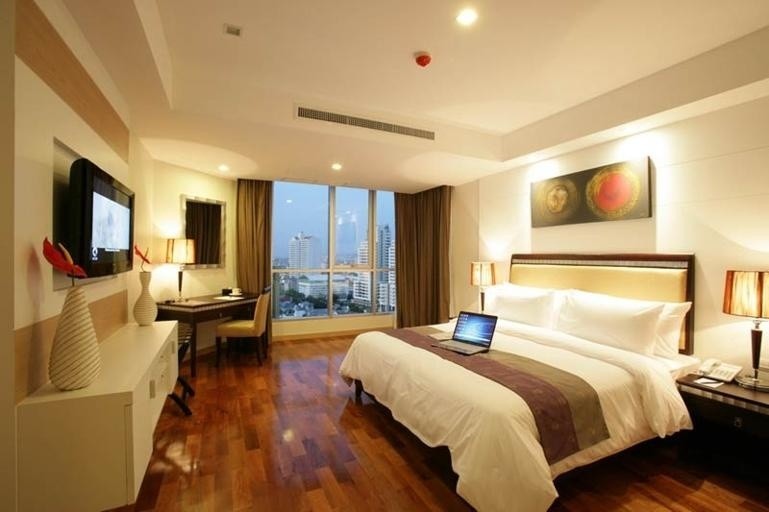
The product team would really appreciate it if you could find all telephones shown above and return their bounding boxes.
[696,358,743,382]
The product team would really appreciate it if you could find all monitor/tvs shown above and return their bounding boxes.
[66,158,135,279]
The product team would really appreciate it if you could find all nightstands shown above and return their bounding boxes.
[677,366,769,492]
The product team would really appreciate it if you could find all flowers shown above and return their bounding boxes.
[134,244,153,272]
[41,236,87,288]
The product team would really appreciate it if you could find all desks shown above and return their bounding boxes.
[156,290,269,377]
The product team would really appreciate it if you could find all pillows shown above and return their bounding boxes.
[498,282,577,330]
[484,285,553,329]
[556,291,666,358]
[653,300,693,358]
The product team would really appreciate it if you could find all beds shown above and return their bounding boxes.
[338,254,695,512]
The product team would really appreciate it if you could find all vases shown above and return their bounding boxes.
[49,286,102,392]
[133,271,158,326]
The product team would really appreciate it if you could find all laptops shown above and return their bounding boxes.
[431,310,498,354]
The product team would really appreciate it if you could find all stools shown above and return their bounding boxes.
[168,326,197,415]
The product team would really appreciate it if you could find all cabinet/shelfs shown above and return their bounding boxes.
[17,317,179,512]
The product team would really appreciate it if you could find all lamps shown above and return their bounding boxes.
[166,238,196,303]
[470,260,495,314]
[723,269,768,390]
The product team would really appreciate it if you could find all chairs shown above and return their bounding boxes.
[213,286,272,368]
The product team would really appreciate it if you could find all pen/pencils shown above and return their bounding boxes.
[701,381,721,384]
